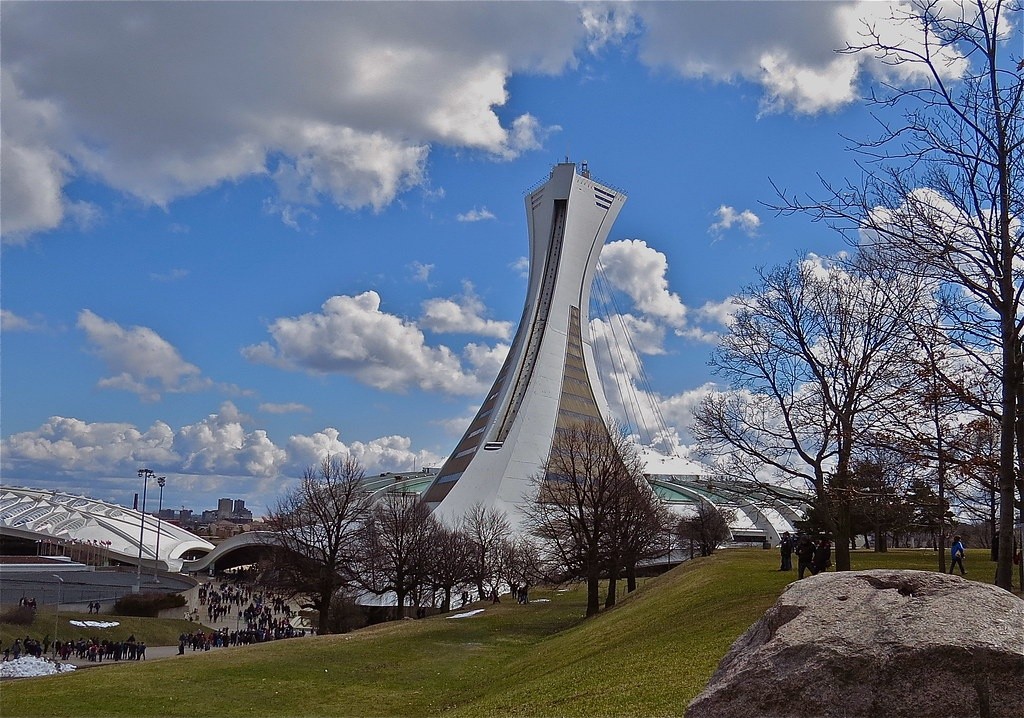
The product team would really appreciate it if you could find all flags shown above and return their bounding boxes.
[35,538,111,546]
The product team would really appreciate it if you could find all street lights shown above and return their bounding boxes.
[136,468,155,587]
[151,476,166,584]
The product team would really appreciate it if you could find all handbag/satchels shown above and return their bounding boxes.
[825,560,832,567]
[955,549,961,558]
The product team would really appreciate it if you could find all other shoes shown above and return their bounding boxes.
[962,572,967,575]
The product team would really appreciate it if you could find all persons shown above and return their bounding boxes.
[19,597,37,611]
[949,535,967,575]
[179,562,314,655]
[493,587,500,604]
[87,601,100,614]
[512,582,528,604]
[780,531,831,580]
[3,634,146,661]
